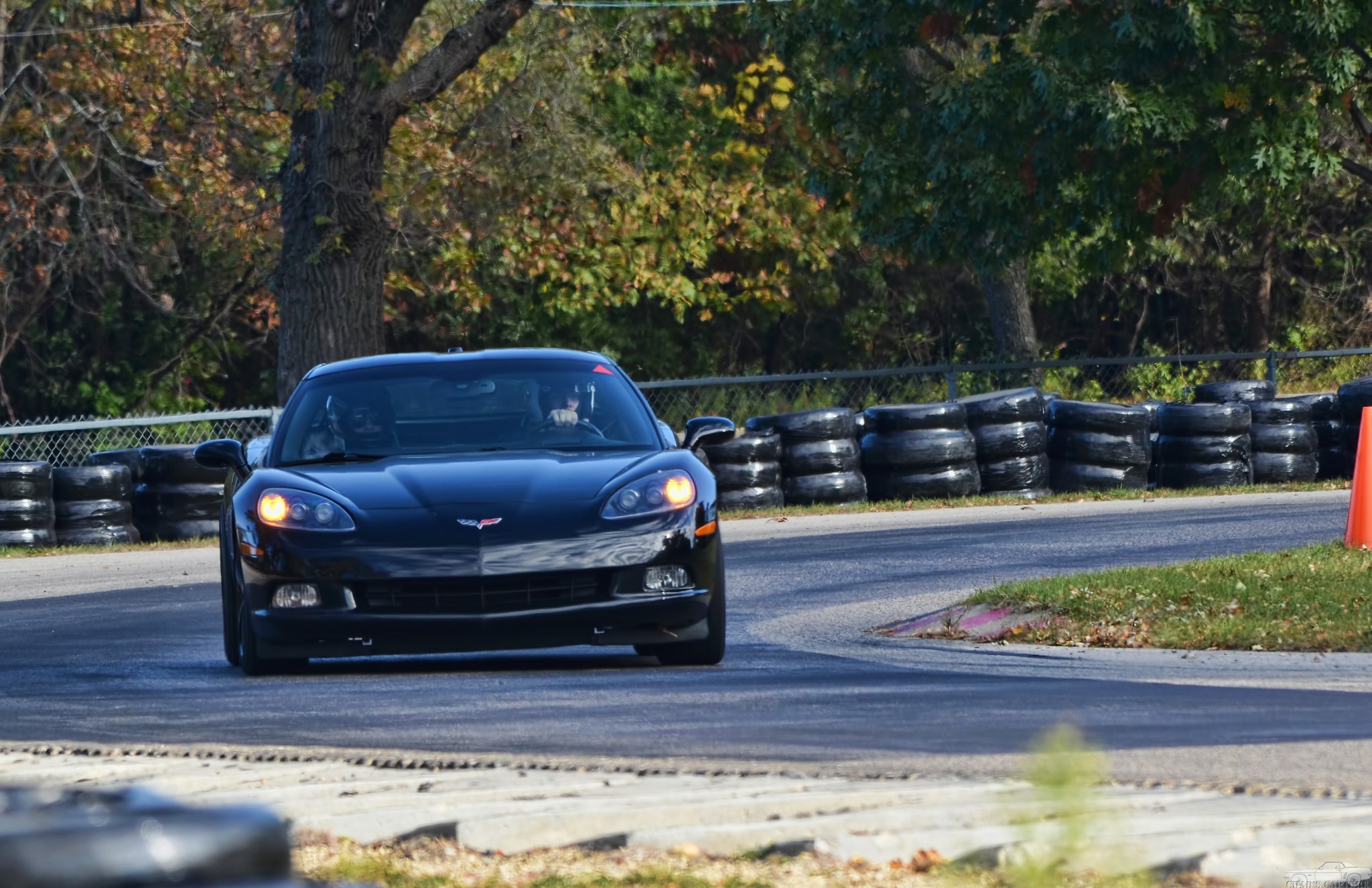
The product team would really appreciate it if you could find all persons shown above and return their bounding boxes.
[314,384,397,452]
[517,376,598,440]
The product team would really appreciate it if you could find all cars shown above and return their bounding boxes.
[194,343,735,668]
